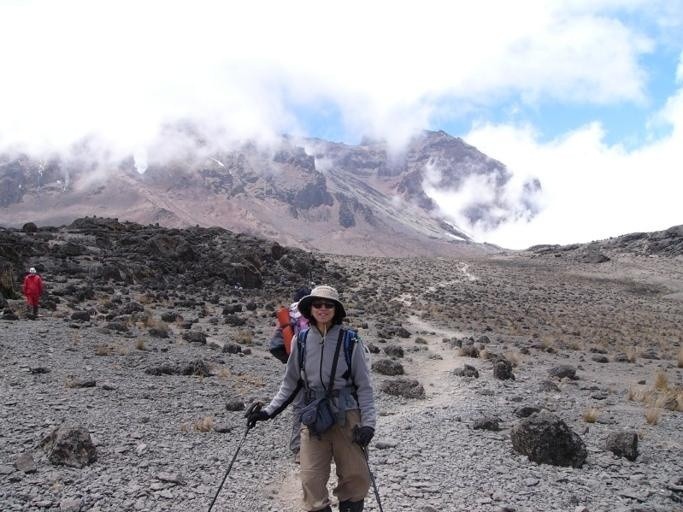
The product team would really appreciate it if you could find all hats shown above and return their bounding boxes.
[296,285,345,324]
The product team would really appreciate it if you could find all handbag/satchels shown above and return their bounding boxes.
[300,398,335,433]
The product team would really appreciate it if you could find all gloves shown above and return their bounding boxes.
[245,410,268,429]
[355,426,374,447]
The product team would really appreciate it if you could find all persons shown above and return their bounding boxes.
[22,267,44,317]
[245,284,376,511]
[269,283,312,463]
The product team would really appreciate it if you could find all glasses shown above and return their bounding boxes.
[310,300,336,309]
